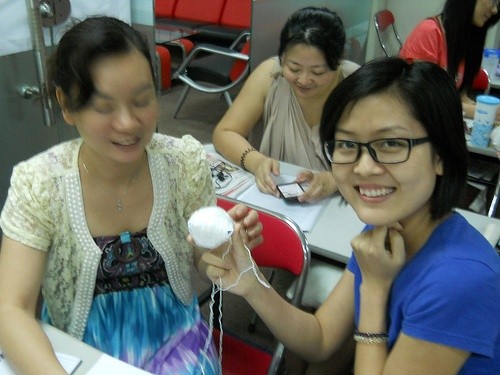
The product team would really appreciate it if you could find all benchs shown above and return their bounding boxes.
[154,0,253,59]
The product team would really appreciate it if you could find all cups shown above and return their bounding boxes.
[483,49,499,81]
[470,95,500,149]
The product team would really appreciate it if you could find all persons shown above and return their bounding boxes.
[0,12,264,375]
[399,0,500,122]
[212,6,362,205]
[188,56,500,375]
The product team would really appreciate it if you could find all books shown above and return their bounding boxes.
[226,174,332,234]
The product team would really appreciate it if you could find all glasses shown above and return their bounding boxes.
[324,137,432,164]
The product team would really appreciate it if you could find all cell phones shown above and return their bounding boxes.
[276,182,305,200]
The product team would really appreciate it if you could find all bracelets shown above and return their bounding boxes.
[240,148,258,171]
[354,330,388,344]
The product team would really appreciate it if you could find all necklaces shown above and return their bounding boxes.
[81,155,146,210]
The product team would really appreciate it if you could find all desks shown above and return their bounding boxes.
[205,142,500,265]
[155,25,208,96]
[462,117,500,187]
[0,321,102,375]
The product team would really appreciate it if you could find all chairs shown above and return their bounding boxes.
[214,191,311,375]
[172,32,252,119]
[373,9,403,58]
